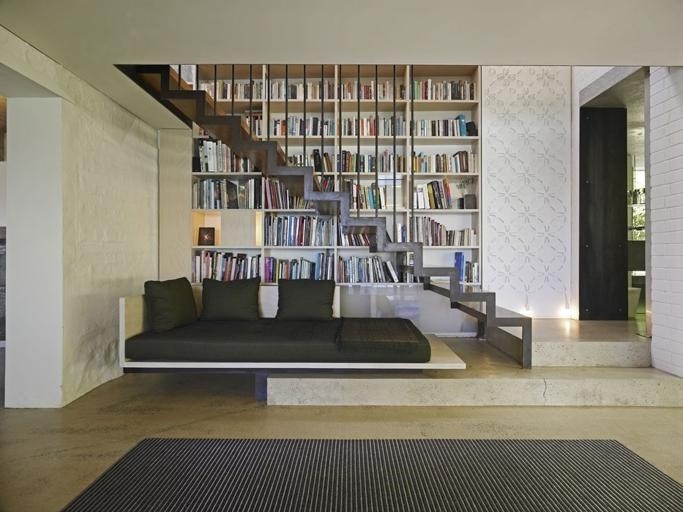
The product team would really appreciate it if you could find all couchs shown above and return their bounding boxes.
[119,289,468,401]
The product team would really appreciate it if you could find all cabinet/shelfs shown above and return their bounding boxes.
[188,63,483,318]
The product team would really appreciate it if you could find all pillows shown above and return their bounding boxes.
[143,276,337,334]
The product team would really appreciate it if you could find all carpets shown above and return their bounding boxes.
[60,436,681,511]
